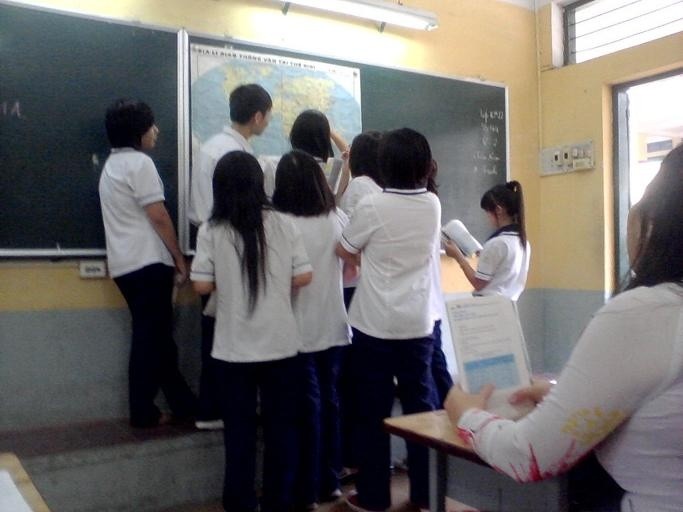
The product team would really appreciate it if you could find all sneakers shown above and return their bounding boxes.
[345,490,371,512]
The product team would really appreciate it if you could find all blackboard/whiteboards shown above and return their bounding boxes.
[0,0,510,257]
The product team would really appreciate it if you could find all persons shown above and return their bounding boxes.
[444,180,532,302]
[97,96,201,431]
[443,138,683,512]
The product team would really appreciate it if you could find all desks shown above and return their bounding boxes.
[378,407,624,510]
[1,450,51,510]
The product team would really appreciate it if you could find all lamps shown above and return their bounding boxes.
[280,0,438,36]
